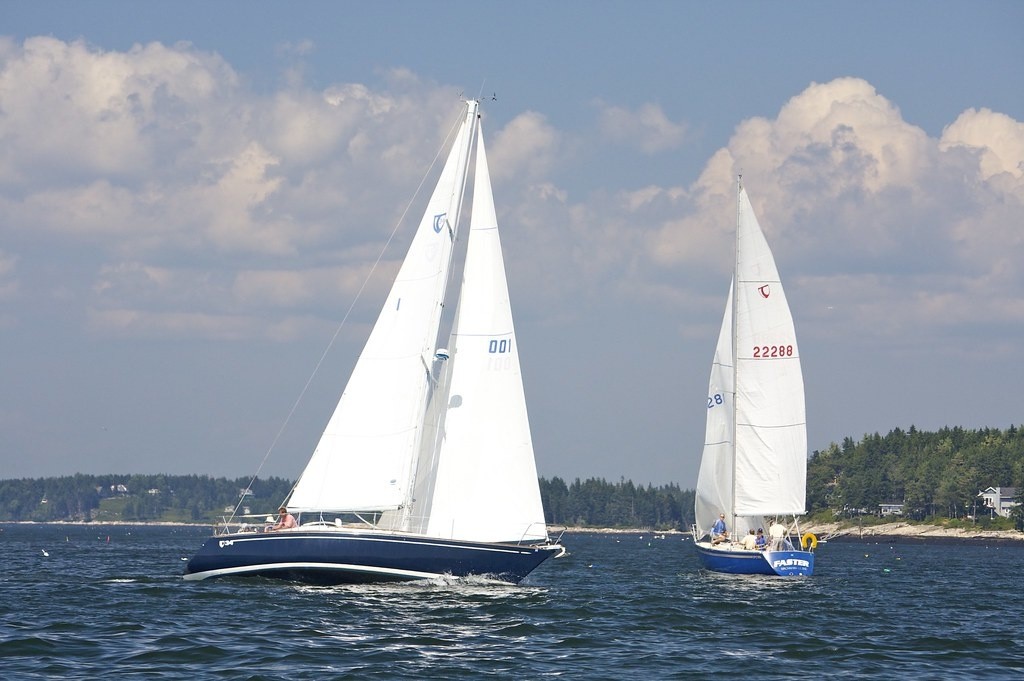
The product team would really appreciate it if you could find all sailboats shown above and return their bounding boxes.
[687,175,819,580]
[181,81,567,587]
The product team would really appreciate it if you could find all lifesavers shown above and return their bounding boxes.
[801,533,817,549]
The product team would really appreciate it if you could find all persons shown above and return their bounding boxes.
[272,508,297,529]
[711,514,727,546]
[770,521,787,551]
[756,528,766,550]
[741,529,757,549]
